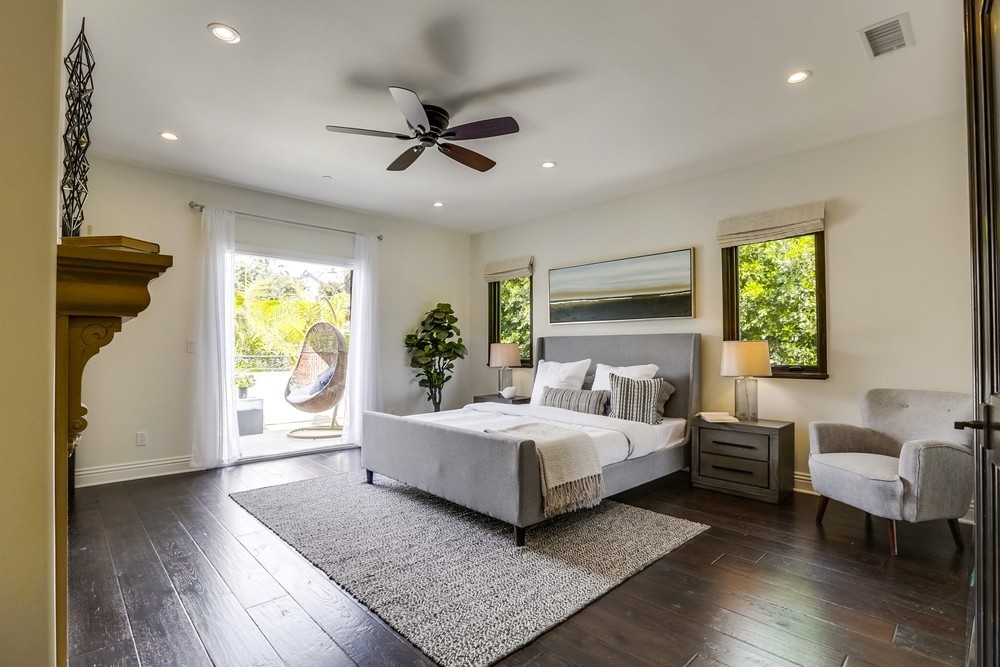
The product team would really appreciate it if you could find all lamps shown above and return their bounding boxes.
[490,343,521,398]
[721,340,772,421]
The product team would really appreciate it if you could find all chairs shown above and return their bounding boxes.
[807,387,976,554]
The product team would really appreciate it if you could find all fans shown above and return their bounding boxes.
[324,86,520,172]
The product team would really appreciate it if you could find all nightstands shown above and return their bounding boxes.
[473,394,531,404]
[689,418,796,504]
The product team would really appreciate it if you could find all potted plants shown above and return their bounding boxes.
[235,373,255,400]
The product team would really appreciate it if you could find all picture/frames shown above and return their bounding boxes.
[546,247,697,326]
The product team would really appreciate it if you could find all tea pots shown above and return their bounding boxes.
[499,386,518,399]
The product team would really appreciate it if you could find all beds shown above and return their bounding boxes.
[362,333,701,547]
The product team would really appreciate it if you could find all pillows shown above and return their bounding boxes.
[530,358,677,426]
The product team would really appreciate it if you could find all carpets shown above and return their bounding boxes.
[227,467,714,667]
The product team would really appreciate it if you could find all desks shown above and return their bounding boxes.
[234,396,263,436]
